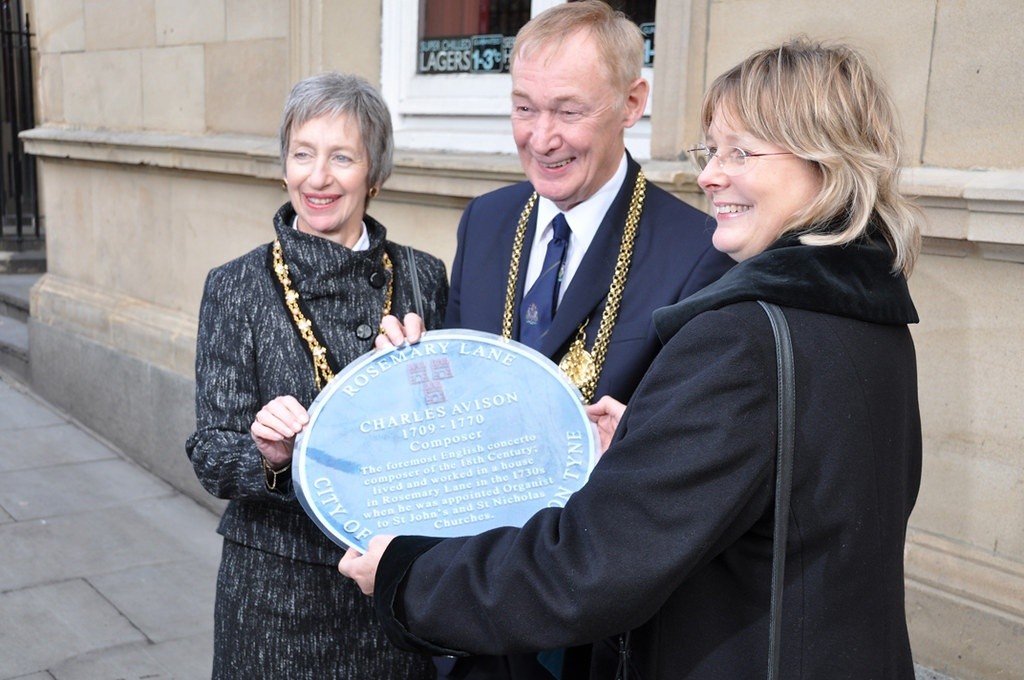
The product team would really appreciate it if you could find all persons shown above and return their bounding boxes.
[337,43,924,680]
[186,74,449,680]
[373,0,738,680]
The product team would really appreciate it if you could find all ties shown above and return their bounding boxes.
[519,213,572,346]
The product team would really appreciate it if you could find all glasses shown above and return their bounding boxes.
[686,143,793,176]
